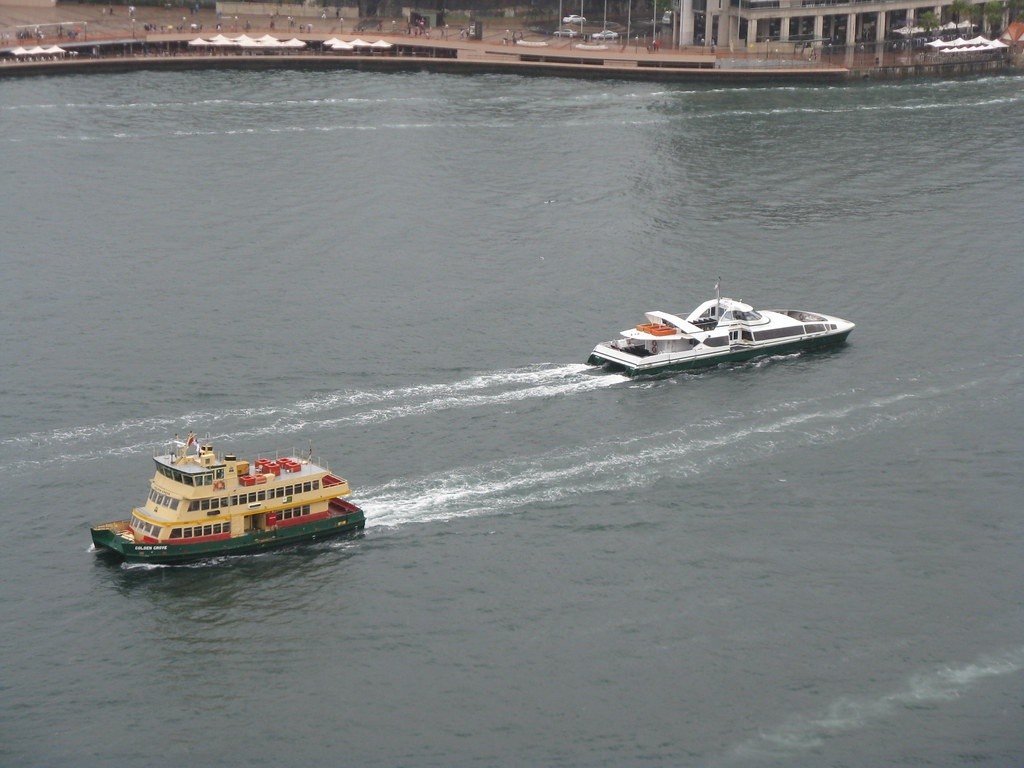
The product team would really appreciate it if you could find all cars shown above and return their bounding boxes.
[552,28,579,39]
[529,22,551,35]
[562,13,587,25]
[592,29,619,40]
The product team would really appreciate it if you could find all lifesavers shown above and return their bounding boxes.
[215,480,225,490]
[652,347,657,353]
[652,340,657,346]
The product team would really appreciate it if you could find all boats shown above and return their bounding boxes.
[88,429,368,566]
[587,274,858,383]
